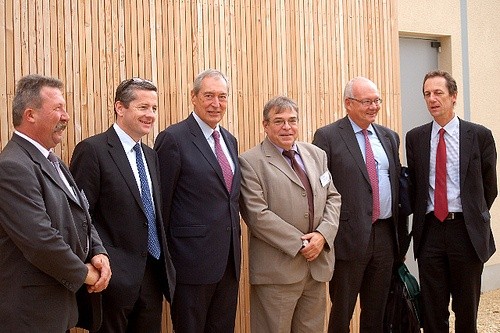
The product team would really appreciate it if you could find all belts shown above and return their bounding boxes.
[431,211,465,220]
[375,218,392,224]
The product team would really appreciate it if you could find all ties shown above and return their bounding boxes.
[360,129,380,224]
[134,143,161,260]
[212,130,233,194]
[434,128,449,223]
[48,152,81,207]
[282,149,313,232]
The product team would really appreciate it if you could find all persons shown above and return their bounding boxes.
[238,97,342,333]
[153,69,242,333]
[312,77,402,333]
[69,77,177,333]
[0,75,112,333]
[406,71,498,333]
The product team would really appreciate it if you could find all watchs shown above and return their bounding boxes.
[302,239,308,248]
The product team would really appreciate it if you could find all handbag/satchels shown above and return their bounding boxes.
[395,263,423,333]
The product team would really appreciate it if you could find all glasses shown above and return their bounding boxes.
[119,78,156,95]
[347,96,382,106]
